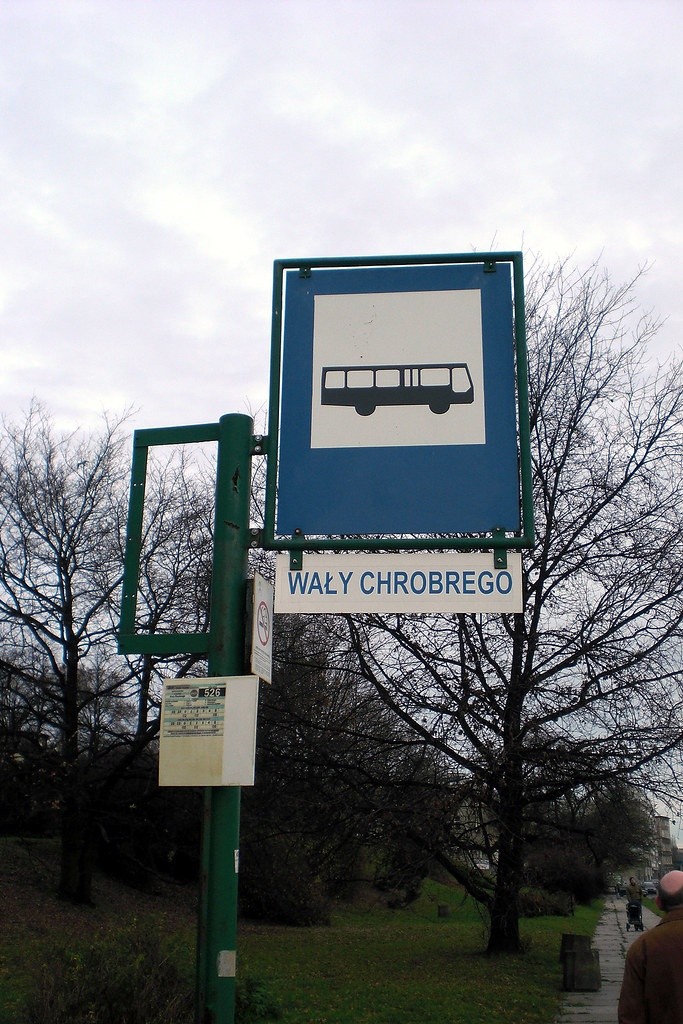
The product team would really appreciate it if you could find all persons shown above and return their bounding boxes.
[618,870,683,1024]
[626,877,643,931]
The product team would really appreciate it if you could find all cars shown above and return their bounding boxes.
[641,878,660,897]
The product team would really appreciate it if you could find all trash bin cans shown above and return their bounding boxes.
[562,948,602,992]
[558,933,590,963]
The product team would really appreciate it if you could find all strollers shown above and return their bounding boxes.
[625,900,643,932]
